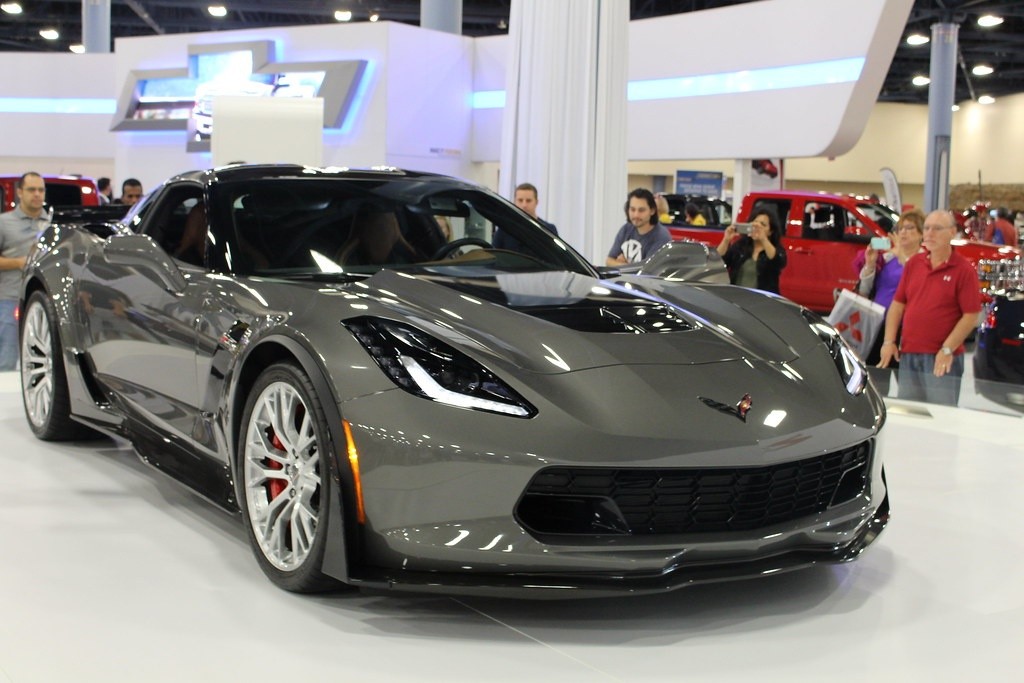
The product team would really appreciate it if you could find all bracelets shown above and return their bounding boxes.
[884,341,896,344]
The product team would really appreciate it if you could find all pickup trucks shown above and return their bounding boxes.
[652,187,1024,383]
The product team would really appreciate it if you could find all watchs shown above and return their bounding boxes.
[941,348,953,355]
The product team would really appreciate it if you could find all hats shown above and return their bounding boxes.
[976,203,989,211]
[998,206,1008,214]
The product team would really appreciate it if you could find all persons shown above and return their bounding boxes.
[492,184,563,265]
[876,210,981,405]
[871,195,894,233]
[985,207,1018,248]
[716,209,787,295]
[97,178,144,204]
[964,205,991,241]
[654,194,706,226]
[854,209,926,396]
[606,188,673,267]
[436,216,464,258]
[0,173,59,372]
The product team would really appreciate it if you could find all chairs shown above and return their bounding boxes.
[177,201,273,271]
[814,208,837,240]
[331,204,422,269]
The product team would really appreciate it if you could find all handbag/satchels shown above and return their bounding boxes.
[992,221,1004,245]
[828,287,886,362]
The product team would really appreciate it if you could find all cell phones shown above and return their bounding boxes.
[871,237,891,249]
[737,223,753,235]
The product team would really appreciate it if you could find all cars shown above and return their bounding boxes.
[751,158,777,177]
[0,173,139,223]
[191,60,318,143]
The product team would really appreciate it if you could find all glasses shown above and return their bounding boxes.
[898,224,915,230]
[127,194,143,198]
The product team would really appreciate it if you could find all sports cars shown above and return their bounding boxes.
[15,159,893,596]
